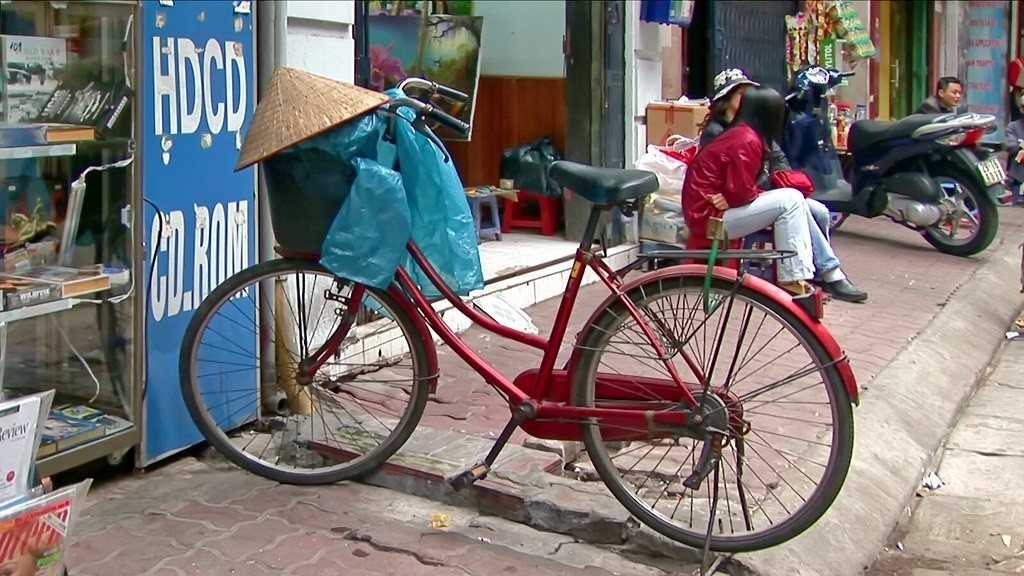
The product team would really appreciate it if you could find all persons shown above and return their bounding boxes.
[1003,94,1024,187]
[682,85,829,305]
[916,76,978,231]
[696,69,867,302]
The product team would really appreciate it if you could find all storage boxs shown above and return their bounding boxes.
[646,102,711,150]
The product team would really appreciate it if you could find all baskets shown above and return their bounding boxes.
[262,147,355,255]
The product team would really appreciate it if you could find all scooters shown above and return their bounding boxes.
[784,63,1009,258]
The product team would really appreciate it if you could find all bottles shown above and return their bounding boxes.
[855,104,866,121]
[831,113,851,148]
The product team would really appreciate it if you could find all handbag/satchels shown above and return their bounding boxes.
[770,169,816,199]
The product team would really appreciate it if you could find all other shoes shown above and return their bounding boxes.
[776,280,832,305]
[960,214,981,225]
[819,278,868,302]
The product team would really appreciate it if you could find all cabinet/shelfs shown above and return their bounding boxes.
[0,138,143,478]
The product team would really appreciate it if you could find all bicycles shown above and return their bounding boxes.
[177,65,861,576]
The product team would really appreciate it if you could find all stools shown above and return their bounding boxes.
[683,237,739,273]
[466,194,503,246]
[504,192,564,237]
[745,229,776,280]
[638,242,684,272]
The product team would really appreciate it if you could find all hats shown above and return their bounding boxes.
[712,68,761,101]
[233,67,391,172]
